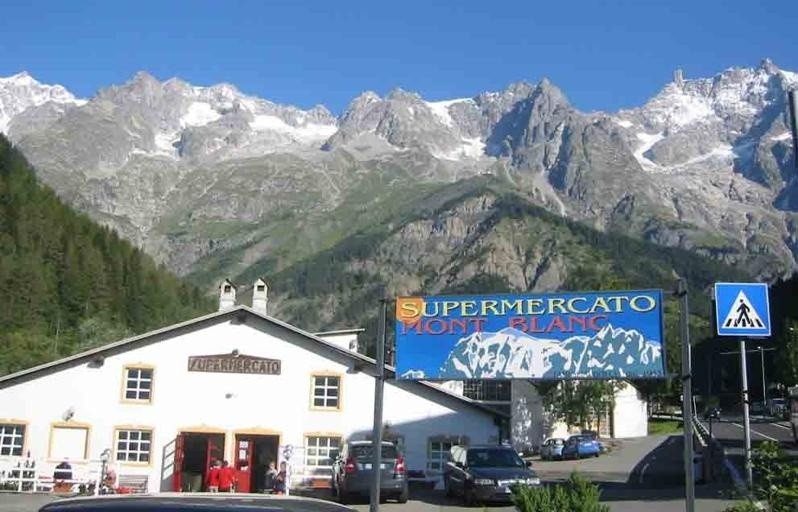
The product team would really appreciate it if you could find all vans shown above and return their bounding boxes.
[442,443,541,506]
[331,440,408,503]
[767,398,789,417]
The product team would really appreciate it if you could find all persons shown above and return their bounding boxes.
[264,462,278,489]
[218,461,234,492]
[54,457,73,491]
[273,462,286,494]
[736,299,750,324]
[106,467,116,485]
[207,465,218,492]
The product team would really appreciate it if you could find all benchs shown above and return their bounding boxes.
[118,473,149,495]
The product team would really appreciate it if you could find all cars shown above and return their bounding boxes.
[750,402,762,415]
[704,408,721,420]
[541,434,601,460]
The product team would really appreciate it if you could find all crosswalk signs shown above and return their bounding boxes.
[715,282,772,337]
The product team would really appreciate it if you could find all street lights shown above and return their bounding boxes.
[757,347,766,406]
[100,452,110,490]
